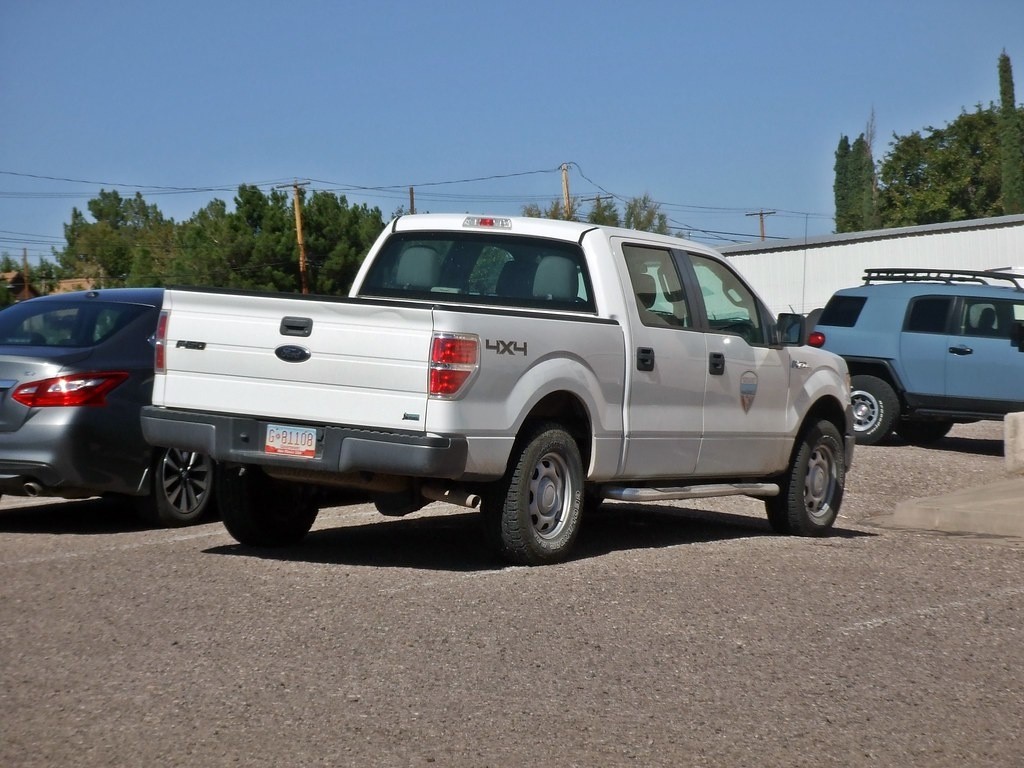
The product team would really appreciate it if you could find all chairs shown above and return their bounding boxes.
[496,257,584,304]
[394,246,440,291]
[633,274,680,324]
[976,308,1000,334]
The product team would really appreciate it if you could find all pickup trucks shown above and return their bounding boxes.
[141,213,855,560]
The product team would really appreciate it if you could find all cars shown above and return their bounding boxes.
[807,268,1024,445]
[0,288,213,524]
